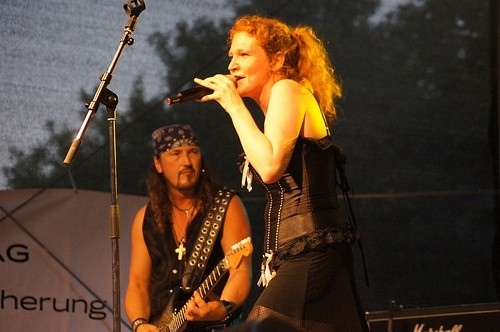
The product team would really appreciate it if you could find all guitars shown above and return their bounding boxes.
[155,236,253,332]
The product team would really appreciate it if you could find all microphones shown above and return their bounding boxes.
[167,86,216,104]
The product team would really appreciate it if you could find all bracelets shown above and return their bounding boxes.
[219,300,232,323]
[132,317,146,330]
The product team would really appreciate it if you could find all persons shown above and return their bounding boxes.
[124,123,253,332]
[194,16,372,332]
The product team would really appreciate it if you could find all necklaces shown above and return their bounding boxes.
[168,195,201,258]
